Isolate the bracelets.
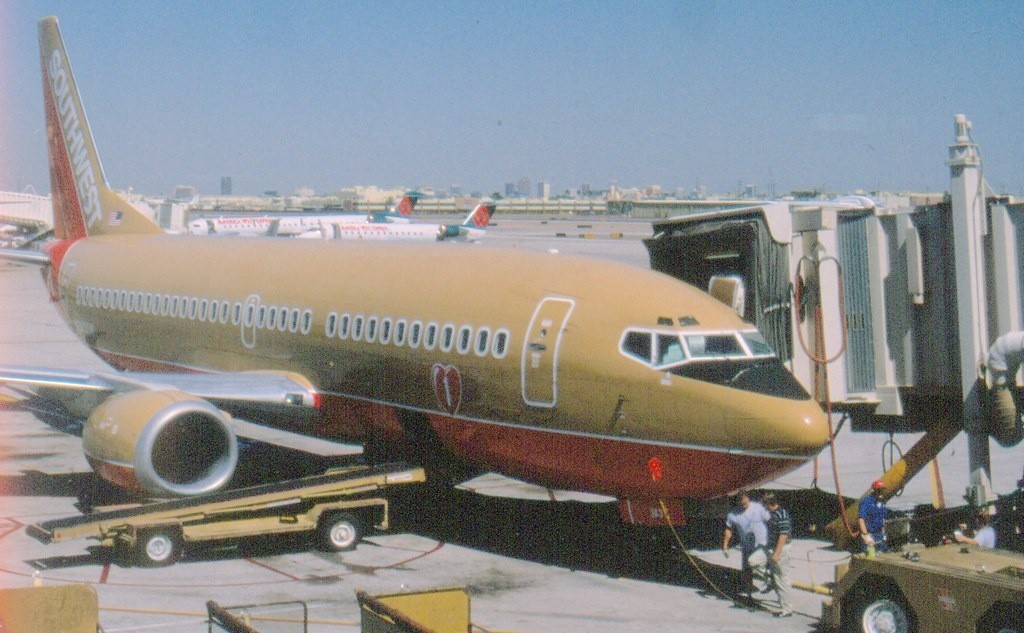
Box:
[773,553,779,556]
[860,532,867,535]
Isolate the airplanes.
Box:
[0,15,829,565]
[187,191,499,243]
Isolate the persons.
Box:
[859,481,888,555]
[76,474,94,514]
[937,504,995,548]
[722,492,792,617]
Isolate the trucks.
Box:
[25,461,426,565]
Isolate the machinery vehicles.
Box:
[813,486,1022,633]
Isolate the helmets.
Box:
[873,480,884,489]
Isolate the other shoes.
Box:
[776,610,790,617]
